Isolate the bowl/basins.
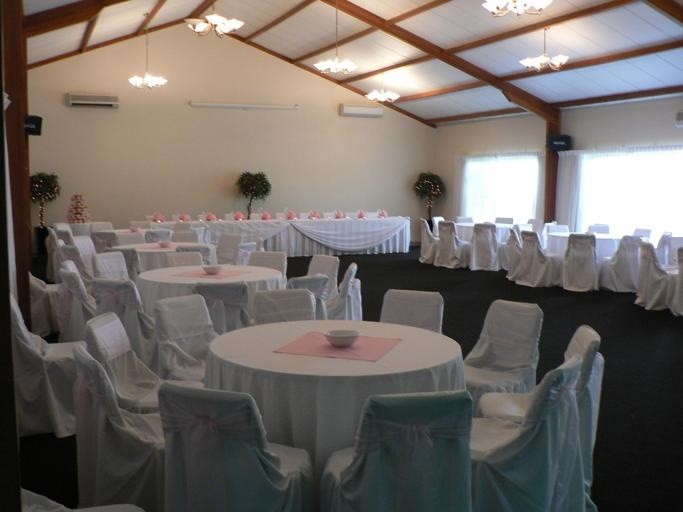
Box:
[324,329,358,347]
[201,264,221,274]
[158,241,170,248]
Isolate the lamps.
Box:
[183,0,246,40]
[518,24,570,74]
[481,1,553,17]
[364,86,400,104]
[311,1,358,77]
[126,11,169,90]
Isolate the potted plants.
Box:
[409,170,447,233]
[27,169,64,259]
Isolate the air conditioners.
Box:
[337,103,383,118]
[65,90,119,111]
